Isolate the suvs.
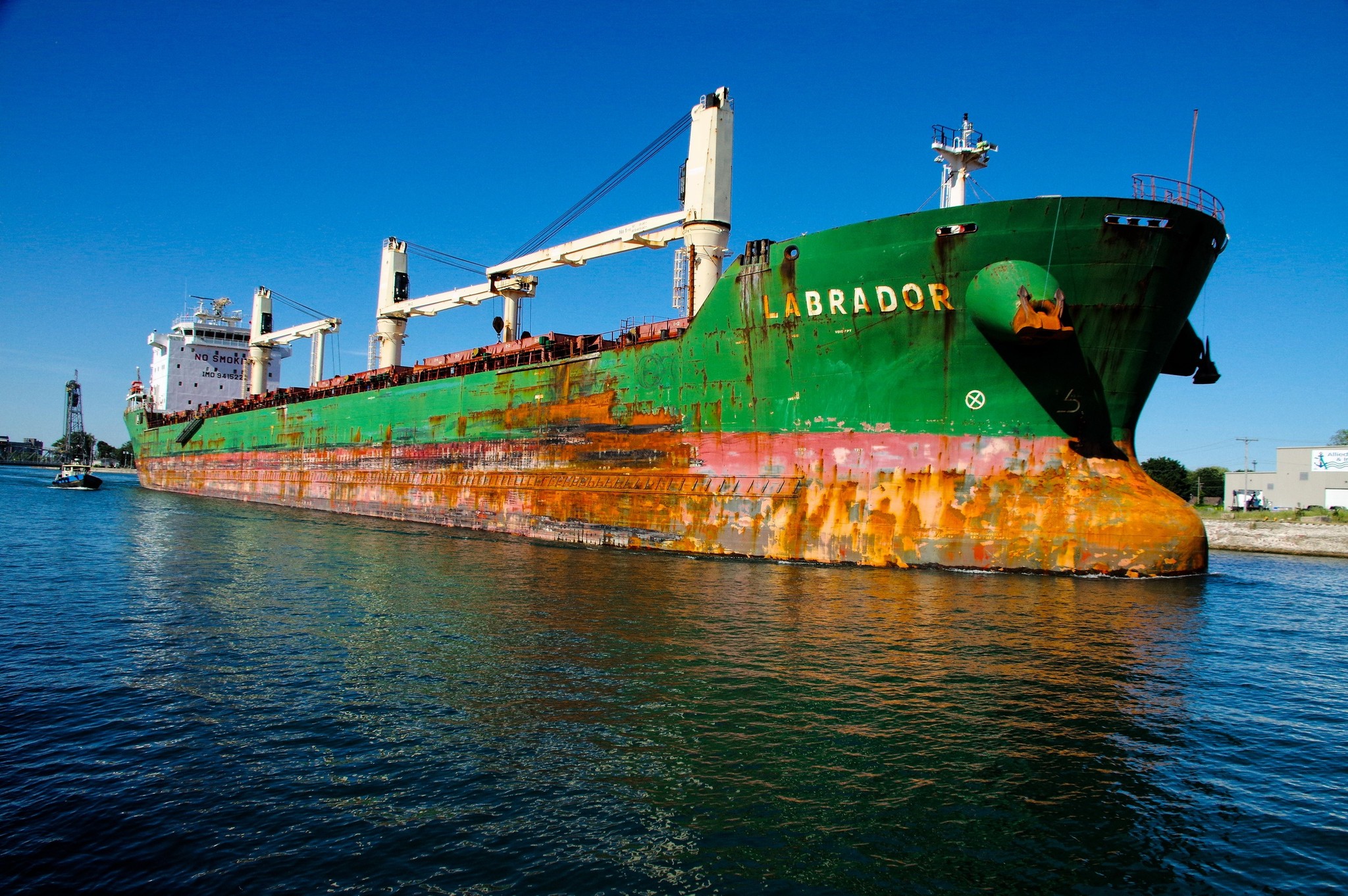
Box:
[1307,505,1347,511]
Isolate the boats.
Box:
[52,459,103,491]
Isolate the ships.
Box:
[121,85,1227,578]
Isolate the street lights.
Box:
[123,450,128,466]
